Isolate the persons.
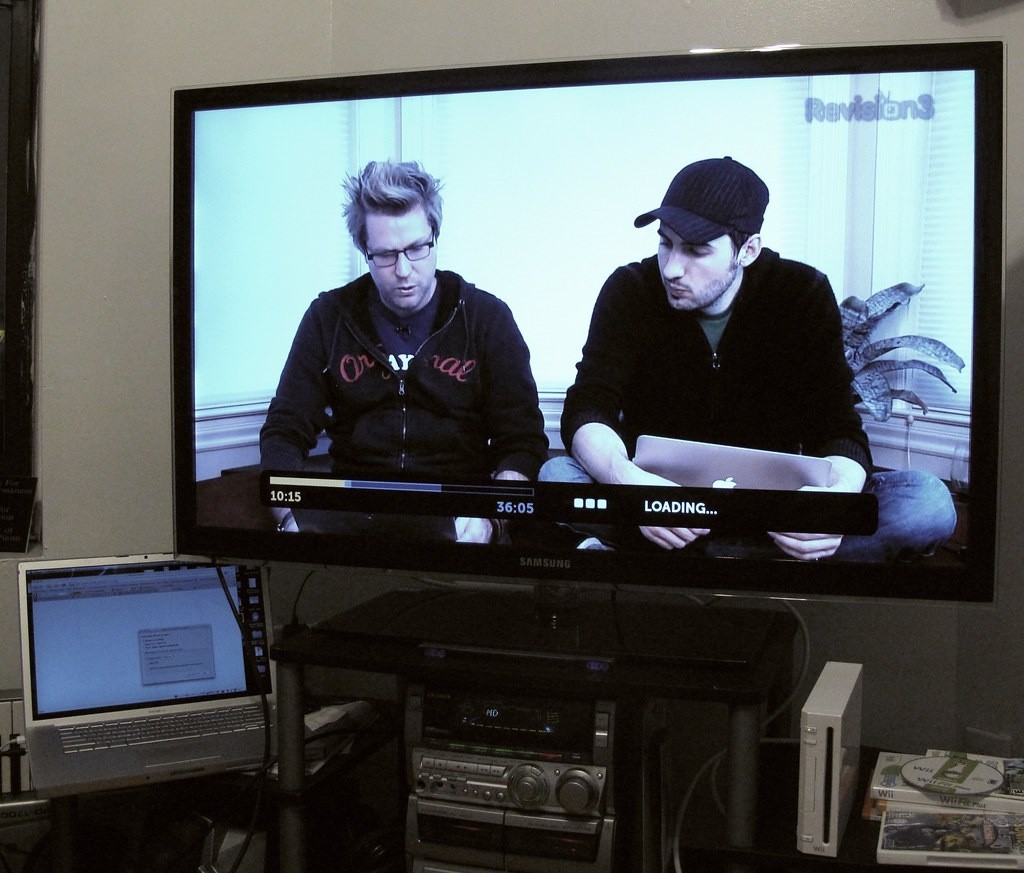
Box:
[259,161,548,544]
[534,156,957,562]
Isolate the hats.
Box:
[634,157,770,244]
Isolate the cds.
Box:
[900,757,1005,795]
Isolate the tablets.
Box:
[633,434,831,492]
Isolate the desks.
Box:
[267,595,781,873]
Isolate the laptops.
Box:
[18,551,325,802]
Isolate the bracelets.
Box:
[490,519,503,543]
[277,511,293,531]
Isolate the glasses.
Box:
[365,227,434,268]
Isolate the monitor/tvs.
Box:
[173,41,1006,665]
